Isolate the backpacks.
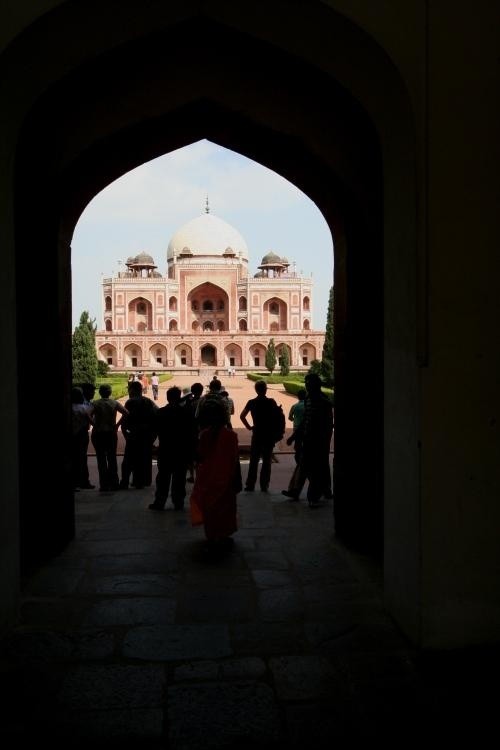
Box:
[259,403,287,444]
